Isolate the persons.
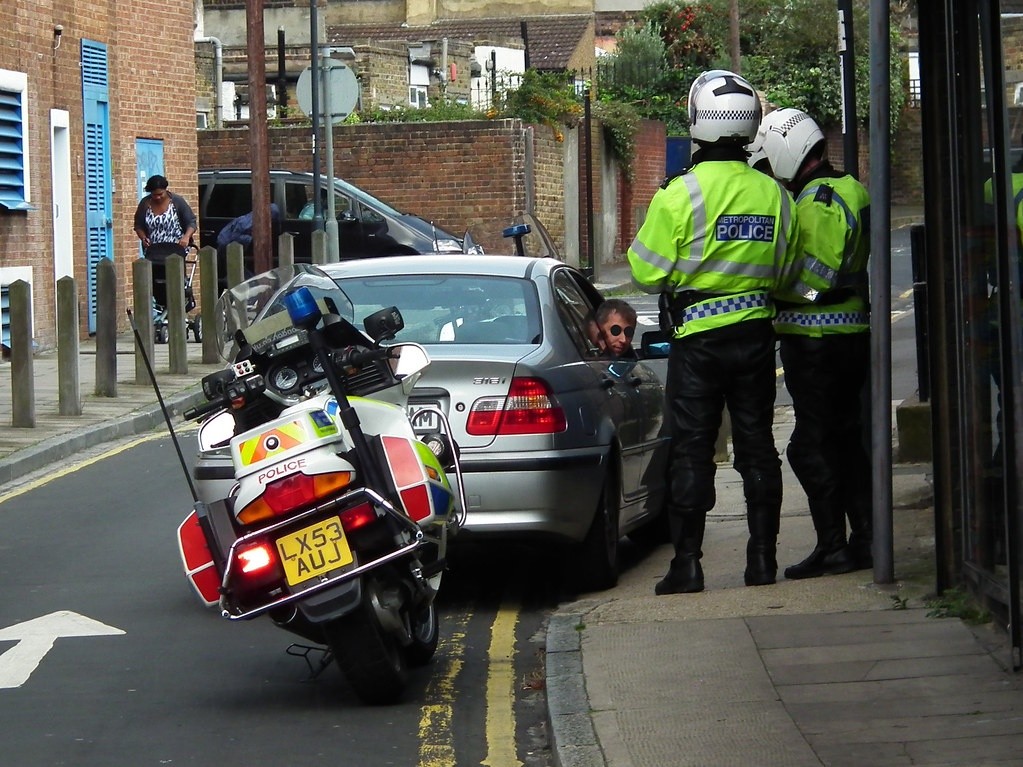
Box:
[983,107,1023,565]
[584,312,610,354]
[298,188,328,219]
[217,204,282,285]
[628,70,803,595]
[134,175,197,339]
[748,104,871,579]
[597,298,638,356]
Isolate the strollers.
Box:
[146,242,202,343]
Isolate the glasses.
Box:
[601,326,636,339]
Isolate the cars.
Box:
[227,255,670,592]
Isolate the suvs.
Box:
[197,167,484,308]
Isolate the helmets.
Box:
[688,70,761,146]
[749,106,826,181]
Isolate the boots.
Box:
[738,472,783,584]
[784,497,852,579]
[844,486,874,570]
[653,506,703,594]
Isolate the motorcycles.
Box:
[178,262,467,706]
[436,213,594,343]
